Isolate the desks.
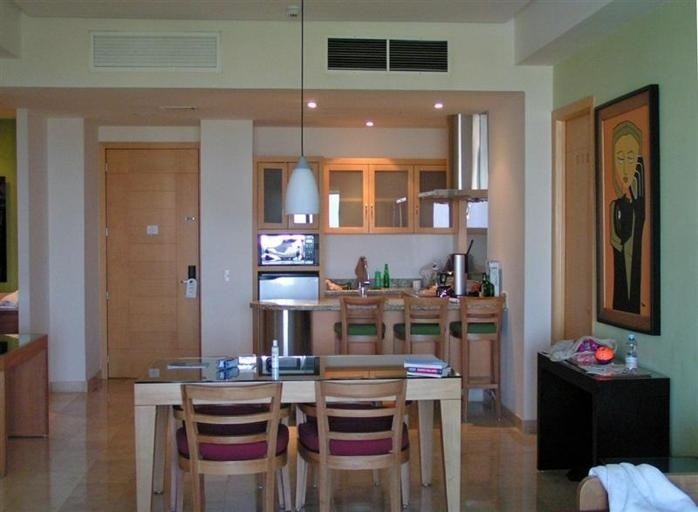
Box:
[3,332,48,479]
[536,349,671,474]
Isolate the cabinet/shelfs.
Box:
[412,160,458,235]
[323,158,414,233]
[254,155,322,230]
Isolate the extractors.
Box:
[434,113,489,203]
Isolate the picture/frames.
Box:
[597,84,659,334]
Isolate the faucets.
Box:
[359,282,370,298]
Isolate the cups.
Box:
[412,280,421,291]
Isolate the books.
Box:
[403,359,449,378]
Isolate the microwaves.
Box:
[256,234,320,267]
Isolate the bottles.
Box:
[271,339,279,380]
[384,263,390,288]
[482,272,488,297]
[624,334,639,369]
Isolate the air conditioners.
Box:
[92,32,229,71]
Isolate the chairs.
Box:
[451,294,508,420]
[298,366,408,512]
[339,292,385,352]
[392,290,451,361]
[172,384,290,512]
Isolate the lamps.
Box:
[282,2,321,216]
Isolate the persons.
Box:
[609,122,644,314]
